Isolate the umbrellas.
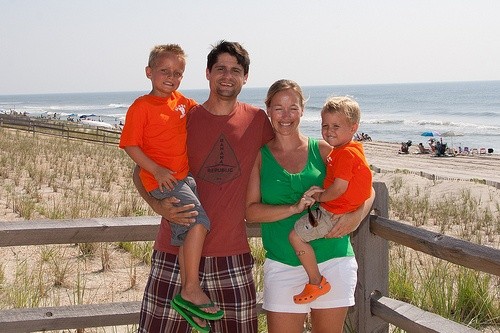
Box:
[421,131,464,147]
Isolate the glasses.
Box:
[307,205,322,227]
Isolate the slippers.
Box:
[174,294,224,320]
[170,300,210,333]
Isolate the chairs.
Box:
[418,143,494,158]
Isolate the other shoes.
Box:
[293,275,331,304]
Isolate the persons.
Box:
[119,43,225,333]
[287,96,372,303]
[138,43,276,333]
[245,79,376,333]
[361,132,442,155]
